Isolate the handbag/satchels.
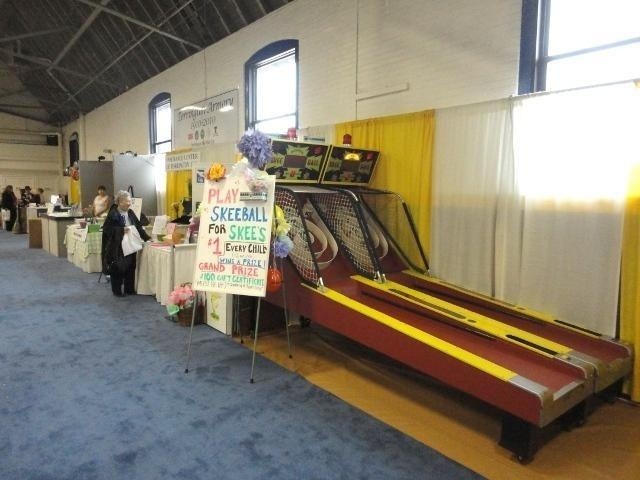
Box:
[121,225,144,257]
[1,209,11,222]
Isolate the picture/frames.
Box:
[173,88,240,150]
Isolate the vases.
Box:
[177,307,201,326]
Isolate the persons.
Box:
[101,203,120,282]
[92,184,110,218]
[1,184,18,234]
[21,185,36,205]
[37,186,47,206]
[102,188,154,298]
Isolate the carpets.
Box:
[0,230,486,480]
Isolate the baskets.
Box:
[176,282,204,327]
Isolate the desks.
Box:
[16,201,198,306]
